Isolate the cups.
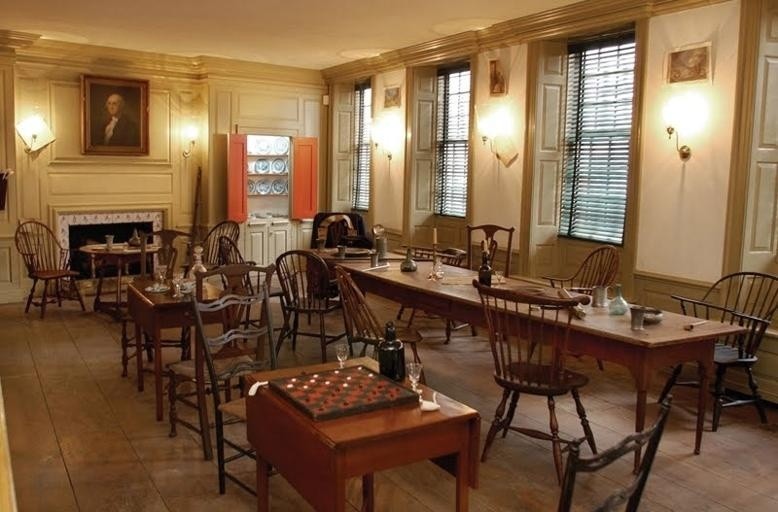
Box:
[370,253,378,268]
[315,238,327,251]
[592,285,608,306]
[376,238,387,257]
[337,245,346,259]
[630,308,645,333]
[105,235,113,250]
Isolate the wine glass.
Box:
[495,271,503,286]
[156,265,167,288]
[335,344,350,369]
[406,362,424,395]
[171,269,185,298]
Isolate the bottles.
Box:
[400,249,417,272]
[607,284,629,316]
[189,245,208,284]
[128,228,142,248]
[479,253,492,287]
[377,322,405,383]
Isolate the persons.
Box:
[97,94,138,146]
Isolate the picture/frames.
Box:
[80,74,148,156]
[662,42,712,87]
[487,57,509,97]
[382,85,403,109]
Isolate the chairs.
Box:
[16,221,86,319]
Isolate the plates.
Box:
[246,137,289,195]
[145,285,171,294]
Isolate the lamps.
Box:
[478,118,503,160]
[662,107,692,162]
[184,127,198,158]
[24,124,41,154]
[371,128,392,160]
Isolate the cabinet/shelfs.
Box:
[226,124,320,277]
